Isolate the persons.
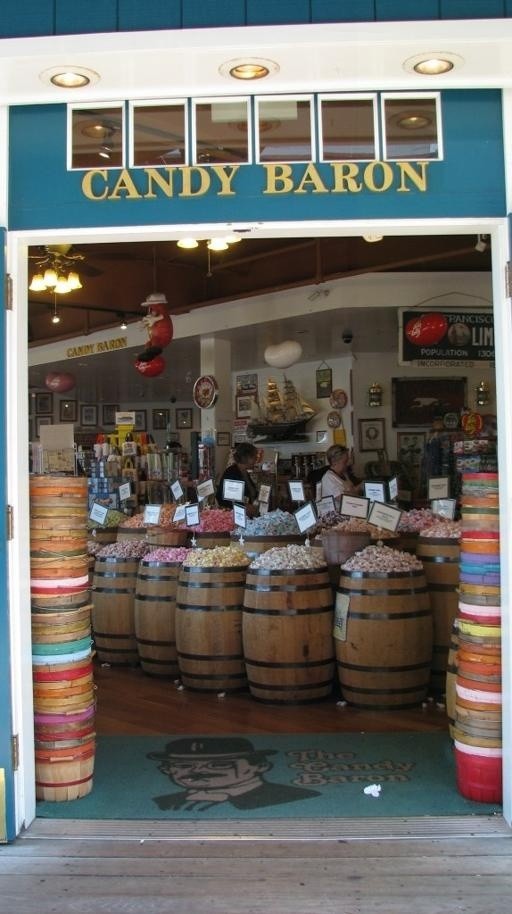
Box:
[215,442,262,520]
[320,444,367,514]
[149,736,324,816]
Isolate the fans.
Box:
[28,244,105,277]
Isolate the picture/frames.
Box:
[358,417,386,452]
[397,431,426,467]
[35,392,192,438]
[393,374,468,428]
[316,431,329,444]
[235,392,256,419]
[217,431,230,446]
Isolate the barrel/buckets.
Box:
[416,533,460,680]
[25,476,99,803]
[114,525,147,542]
[456,471,502,802]
[89,555,142,668]
[147,527,185,553]
[240,567,337,701]
[87,528,113,544]
[172,565,248,693]
[330,568,433,706]
[444,617,455,742]
[320,531,370,566]
[232,532,307,557]
[186,531,231,550]
[131,560,181,677]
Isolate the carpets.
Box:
[35,726,502,819]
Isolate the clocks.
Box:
[193,375,216,409]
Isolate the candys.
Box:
[85,502,462,574]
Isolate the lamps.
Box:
[475,380,489,406]
[29,259,83,325]
[367,382,383,407]
[120,322,127,330]
[176,238,242,277]
